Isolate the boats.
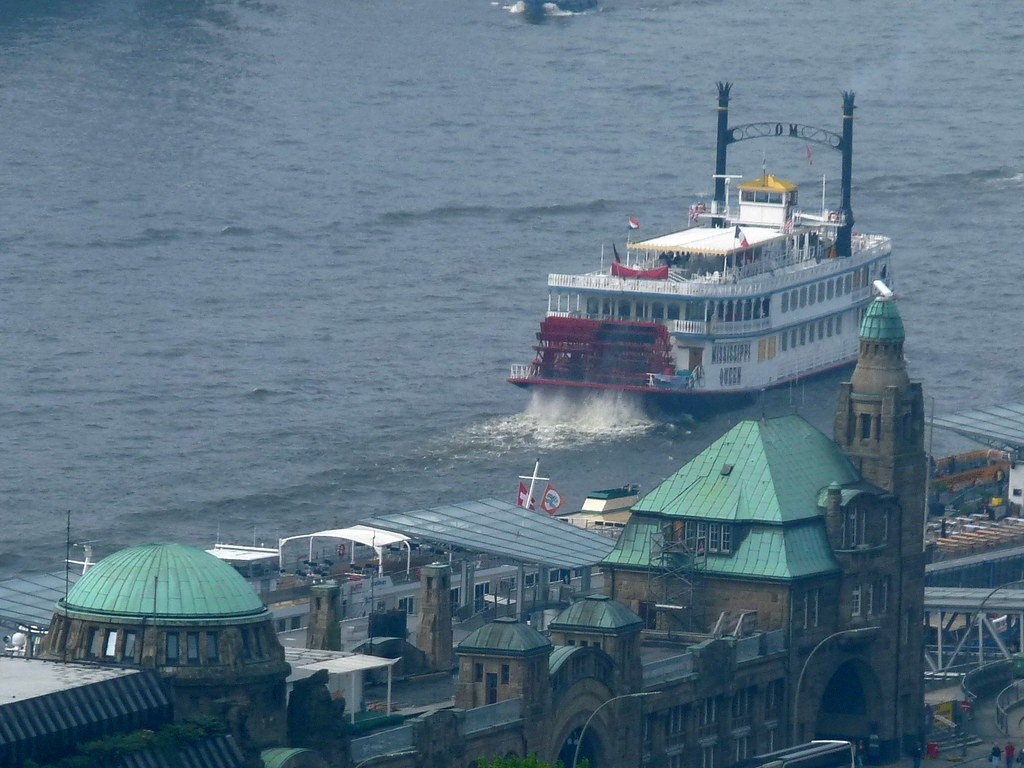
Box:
[521,0,597,26]
[503,75,898,404]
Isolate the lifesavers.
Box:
[696,202,706,214]
[828,211,840,223]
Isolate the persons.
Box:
[990,741,1016,768]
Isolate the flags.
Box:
[783,216,793,235]
[734,224,750,248]
[516,480,565,516]
[628,216,639,229]
